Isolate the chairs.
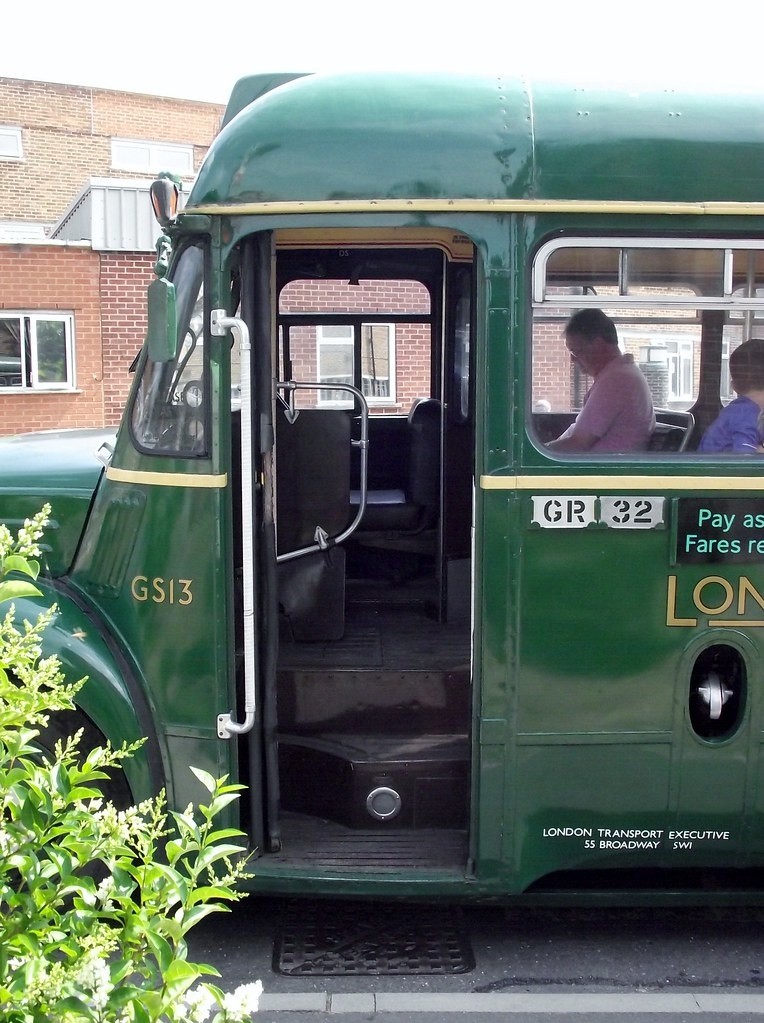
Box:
[649,407,696,452]
[351,397,448,621]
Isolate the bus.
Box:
[0,72,764,902]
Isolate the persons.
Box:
[536,400,551,412]
[544,308,656,453]
[697,339,764,453]
